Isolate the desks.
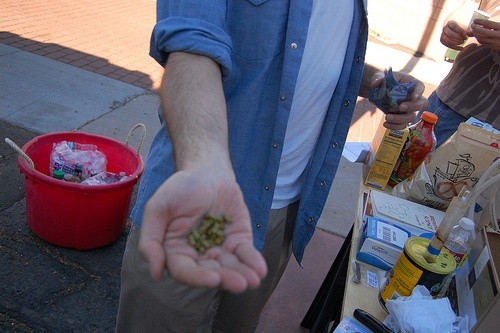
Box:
[301,170,394,333]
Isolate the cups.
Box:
[467,9,490,32]
[444,32,468,64]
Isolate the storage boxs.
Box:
[358,215,415,272]
[362,188,446,237]
[362,114,414,191]
[455,222,500,332]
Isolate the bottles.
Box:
[52,170,64,182]
[388,111,438,187]
[116,172,125,183]
[437,217,475,299]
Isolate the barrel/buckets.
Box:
[5,122,146,248]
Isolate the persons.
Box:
[415,0,500,150]
[117,0,429,333]
